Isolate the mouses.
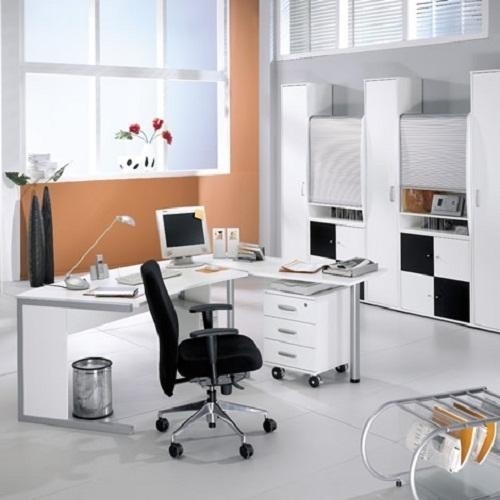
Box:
[205,265,219,271]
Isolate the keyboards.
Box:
[116,266,183,286]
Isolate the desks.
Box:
[17,254,377,434]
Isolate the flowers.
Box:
[115,117,173,145]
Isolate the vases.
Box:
[128,145,164,170]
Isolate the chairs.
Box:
[140,260,278,461]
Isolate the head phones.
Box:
[154,205,213,269]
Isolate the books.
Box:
[238,243,265,261]
[407,402,497,474]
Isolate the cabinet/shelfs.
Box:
[262,285,357,388]
[280,70,500,336]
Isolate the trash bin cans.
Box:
[72,357,113,420]
